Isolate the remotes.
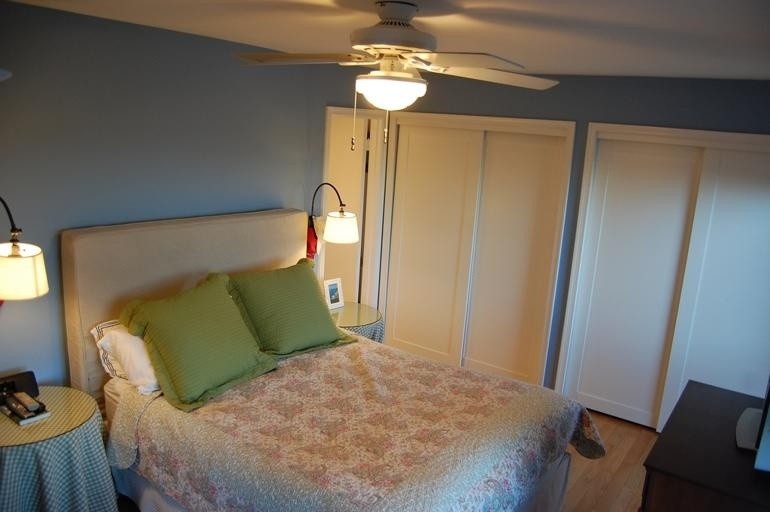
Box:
[14,392,40,411]
[6,397,35,418]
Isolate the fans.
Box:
[231,1,559,91]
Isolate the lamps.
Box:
[354,69,428,112]
[0,239,50,303]
[311,180,361,247]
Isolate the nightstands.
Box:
[330,303,384,344]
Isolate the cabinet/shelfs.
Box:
[557,113,761,430]
[378,105,570,388]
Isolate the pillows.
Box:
[114,272,282,408]
[91,318,162,395]
[230,259,359,361]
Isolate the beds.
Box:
[57,207,595,512]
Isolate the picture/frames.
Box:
[323,276,345,310]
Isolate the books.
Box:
[1,397,52,426]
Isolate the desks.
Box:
[639,379,770,511]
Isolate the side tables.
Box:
[1,382,123,512]
